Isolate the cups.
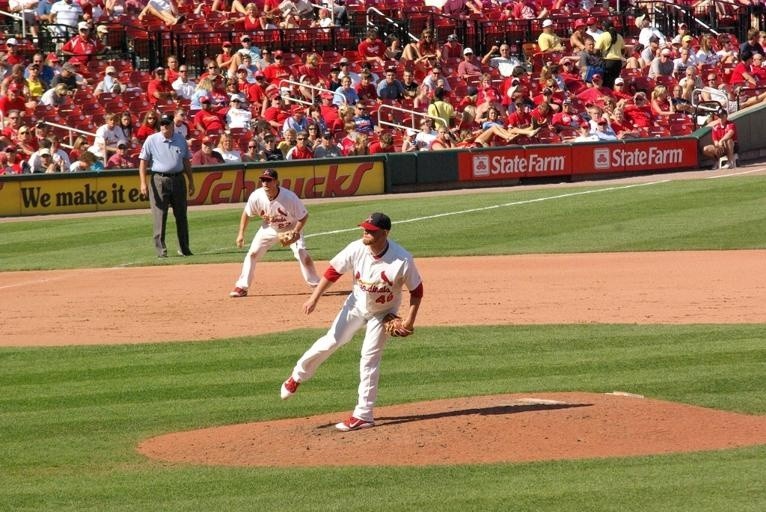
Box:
[411,141,416,145]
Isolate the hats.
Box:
[5,21,741,158]
[357,211,391,232]
[259,168,278,181]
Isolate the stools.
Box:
[718,153,739,168]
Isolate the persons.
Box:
[230,169,326,296]
[279,212,423,431]
[1,1,766,177]
[136,113,195,257]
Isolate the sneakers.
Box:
[228,286,248,298]
[280,375,301,400]
[333,415,376,433]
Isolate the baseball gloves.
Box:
[278,230,299,248]
[382,312,413,338]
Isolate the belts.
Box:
[155,172,183,177]
